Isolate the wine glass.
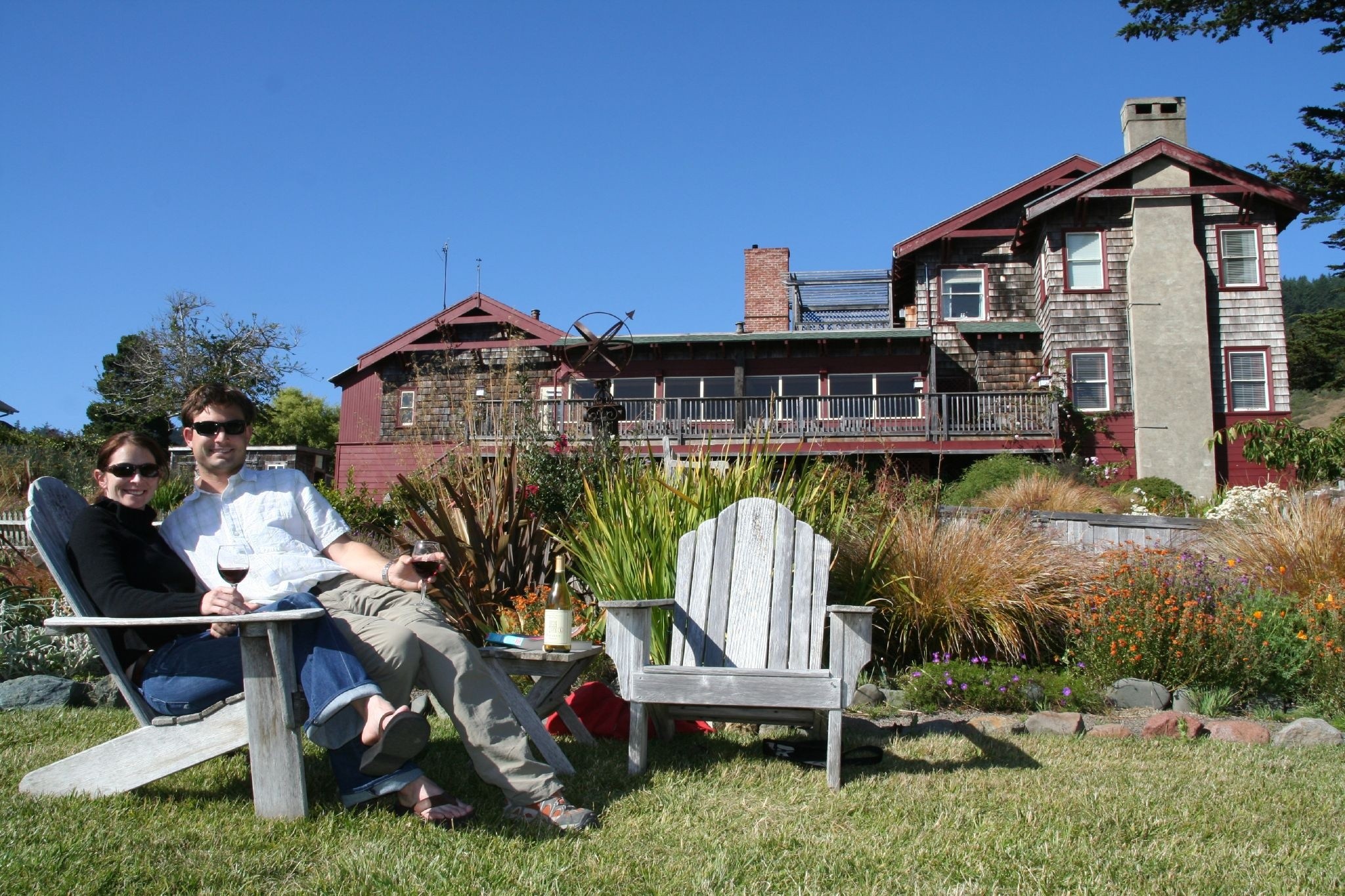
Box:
[217,544,249,589]
[409,541,440,606]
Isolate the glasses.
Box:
[102,463,160,478]
[191,419,249,436]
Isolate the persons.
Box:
[158,382,594,832]
[65,429,475,823]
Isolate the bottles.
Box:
[543,555,572,653]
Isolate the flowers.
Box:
[1028,372,1041,383]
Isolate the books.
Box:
[485,623,593,650]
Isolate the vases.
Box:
[1031,381,1040,389]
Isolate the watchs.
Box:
[382,562,394,588]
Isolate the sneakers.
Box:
[502,790,600,835]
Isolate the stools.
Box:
[477,642,602,775]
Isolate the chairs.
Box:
[19,476,325,820]
[599,498,875,793]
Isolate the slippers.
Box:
[357,710,430,778]
[394,790,477,823]
